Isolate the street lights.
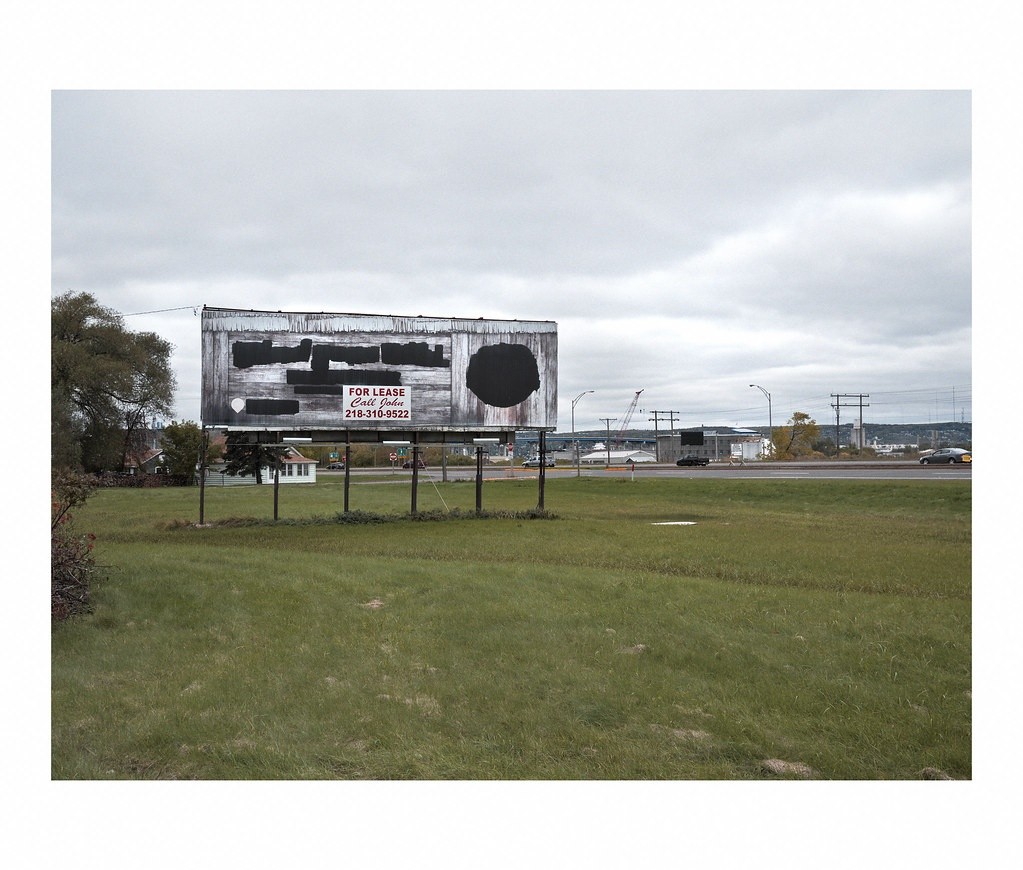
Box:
[571,390,595,467]
[749,384,772,456]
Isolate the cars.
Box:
[521,456,555,468]
[326,462,345,469]
[919,447,972,464]
[402,459,425,469]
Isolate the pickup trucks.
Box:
[676,455,709,466]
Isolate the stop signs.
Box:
[390,452,397,461]
